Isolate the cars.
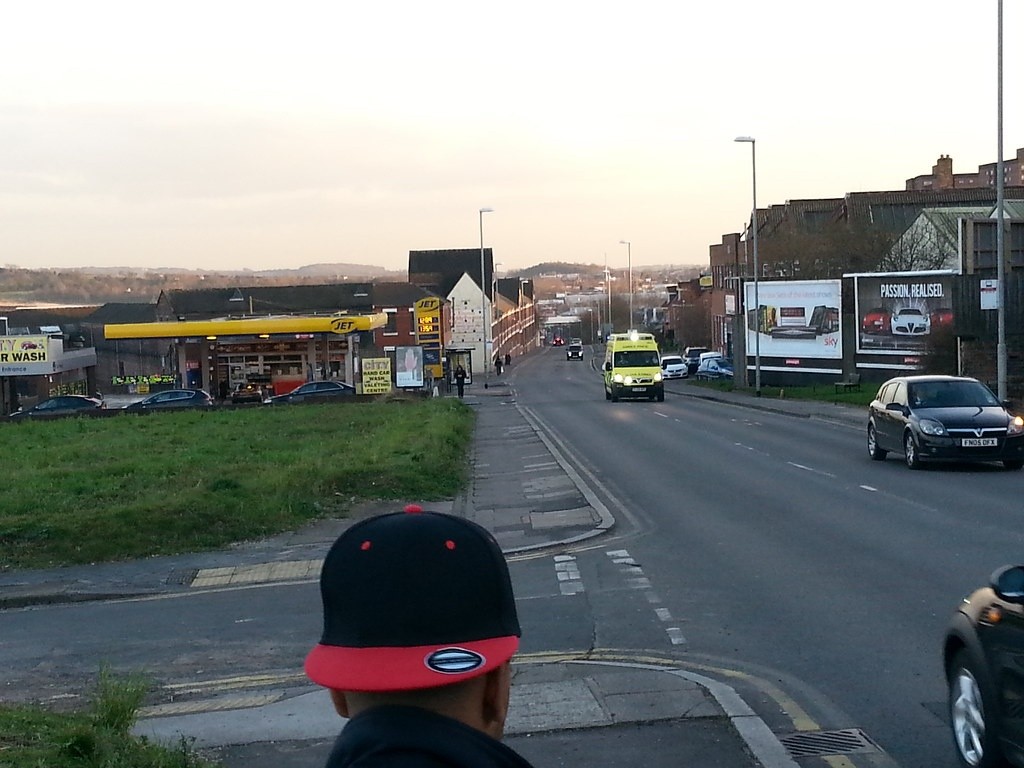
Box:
[694,351,733,383]
[264,380,382,406]
[943,563,1024,768]
[867,374,1024,469]
[553,338,564,346]
[863,308,954,335]
[122,388,214,410]
[661,356,688,379]
[10,394,107,418]
[572,338,582,345]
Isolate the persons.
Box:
[455,365,465,398]
[618,355,631,365]
[303,505,530,768]
[495,356,502,375]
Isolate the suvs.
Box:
[566,345,584,360]
[682,347,710,371]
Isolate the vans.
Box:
[603,332,667,402]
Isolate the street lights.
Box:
[620,240,633,332]
[495,262,503,358]
[596,301,601,345]
[480,207,494,389]
[521,280,529,355]
[602,270,612,335]
[735,135,761,397]
[588,309,593,344]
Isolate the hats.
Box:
[303,504,520,690]
